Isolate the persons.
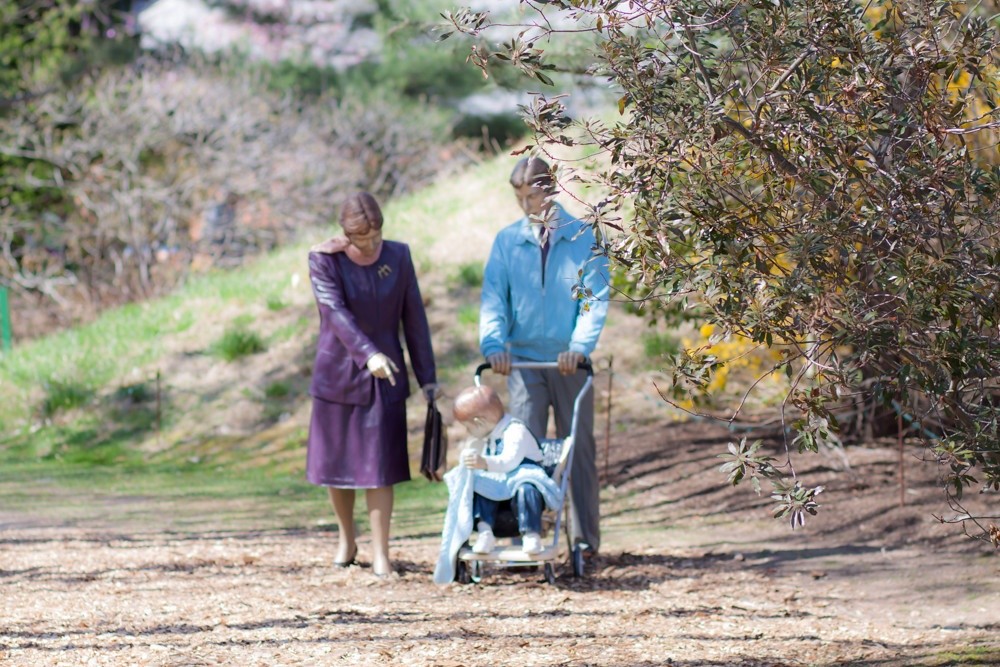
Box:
[305,192,439,578]
[453,385,548,556]
[478,155,611,568]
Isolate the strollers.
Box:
[455,362,594,584]
